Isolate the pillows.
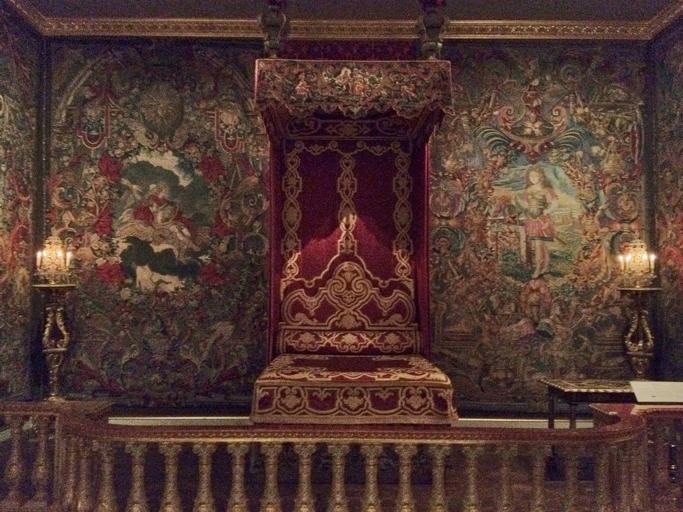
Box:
[275,329,421,356]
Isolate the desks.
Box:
[68,400,115,427]
[537,375,648,428]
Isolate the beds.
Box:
[249,58,462,426]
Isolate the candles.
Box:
[36,249,72,267]
[619,255,657,269]
